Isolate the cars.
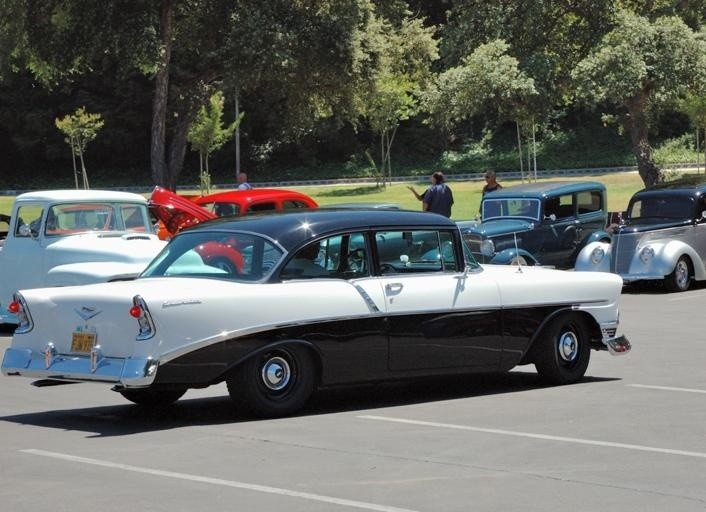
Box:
[0,193,205,241]
[237,202,478,278]
[574,176,706,292]
[150,185,319,274]
[0,188,231,325]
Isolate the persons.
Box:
[406,184,430,211]
[479,170,509,216]
[426,170,454,217]
[237,172,250,190]
[284,240,328,277]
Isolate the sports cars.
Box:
[0,207,632,417]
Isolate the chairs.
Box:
[278,237,320,275]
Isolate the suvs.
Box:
[422,181,612,272]
